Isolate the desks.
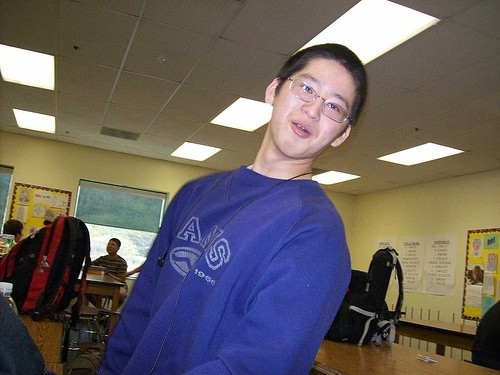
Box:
[74,270,127,341]
[394,318,478,356]
[19,312,66,375]
[312,339,500,375]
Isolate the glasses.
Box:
[288,76,356,125]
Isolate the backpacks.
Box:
[323,246,404,346]
[0,215,92,326]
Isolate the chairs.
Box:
[57,286,128,375]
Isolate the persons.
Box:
[125,264,143,276]
[84,238,129,312]
[2,219,24,246]
[94,44,368,375]
[471,299,500,370]
